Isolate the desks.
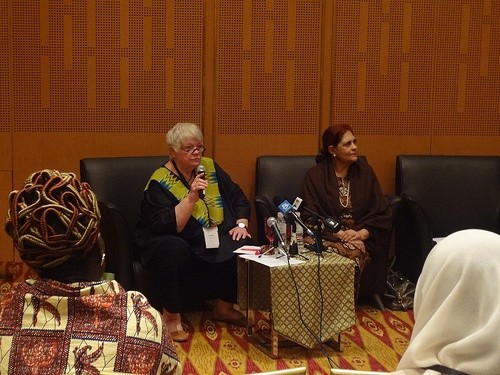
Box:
[244,248,356,359]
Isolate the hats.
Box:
[4,168,102,271]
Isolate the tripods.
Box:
[258,215,332,260]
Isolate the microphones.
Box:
[197,166,206,199]
[267,193,341,253]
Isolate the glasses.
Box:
[181,144,207,154]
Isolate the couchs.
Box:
[395,155,500,282]
[254,155,402,304]
[79,155,221,312]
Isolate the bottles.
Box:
[290,212,304,254]
[275,212,287,250]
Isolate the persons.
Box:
[393,230,500,375]
[300,124,393,308]
[0,169,183,375]
[135,123,252,328]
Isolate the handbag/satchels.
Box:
[383,255,416,309]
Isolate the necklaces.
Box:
[336,170,350,207]
[189,171,194,183]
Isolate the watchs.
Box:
[235,223,248,231]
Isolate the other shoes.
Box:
[169,328,188,341]
[213,307,247,327]
